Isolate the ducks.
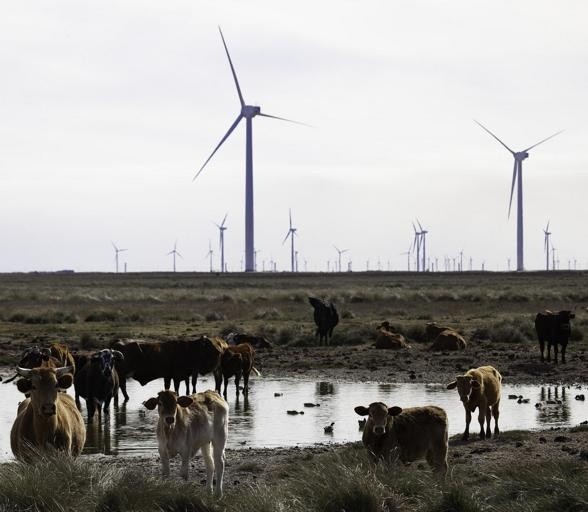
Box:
[323,422,335,433]
[516,395,530,403]
[574,393,584,400]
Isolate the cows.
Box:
[143,389,231,502]
[10,364,87,465]
[308,297,339,346]
[375,321,467,352]
[354,402,449,488]
[2,335,261,425]
[446,365,502,441]
[534,310,575,366]
